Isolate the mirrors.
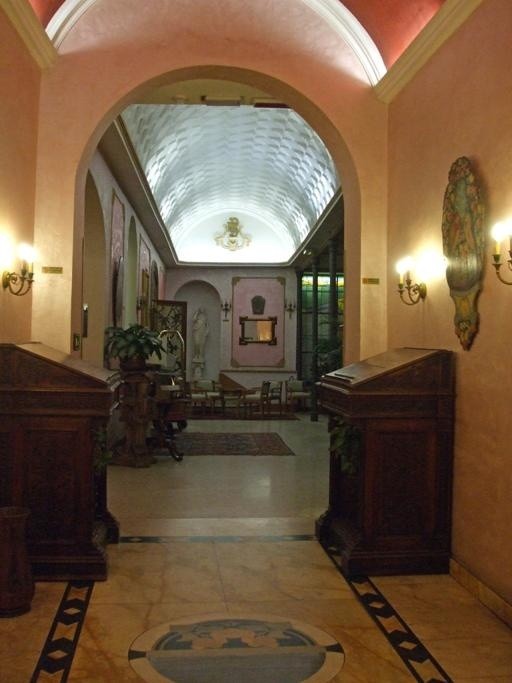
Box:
[238,315,278,345]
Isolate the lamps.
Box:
[284,295,296,317]
[3,242,36,297]
[492,219,511,285]
[214,216,252,250]
[396,259,426,305]
[221,296,232,321]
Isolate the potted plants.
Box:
[106,325,166,372]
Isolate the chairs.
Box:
[171,372,315,421]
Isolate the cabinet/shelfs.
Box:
[0,339,127,582]
[315,343,457,582]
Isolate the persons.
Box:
[191,305,210,360]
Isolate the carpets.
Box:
[157,431,295,456]
